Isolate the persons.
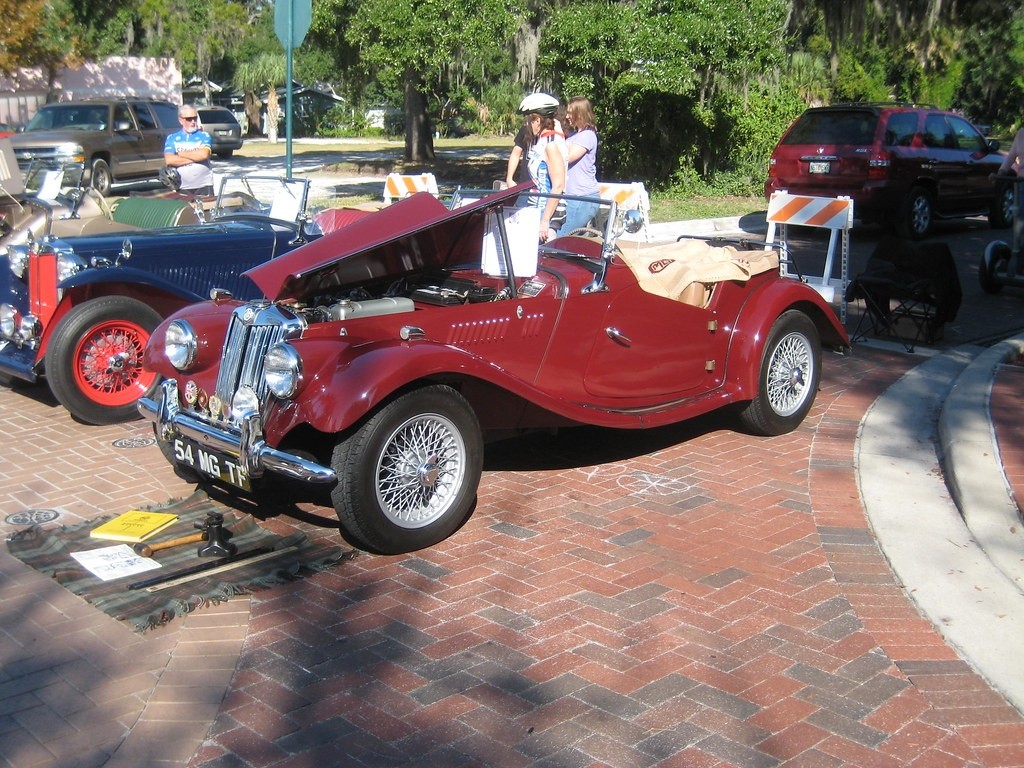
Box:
[989,126,1024,276]
[507,93,600,245]
[164,105,215,197]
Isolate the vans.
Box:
[192,105,245,157]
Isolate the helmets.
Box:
[516,93,561,117]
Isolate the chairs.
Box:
[613,239,717,302]
[108,196,200,232]
[849,237,951,354]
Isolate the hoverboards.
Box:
[978,174,1023,293]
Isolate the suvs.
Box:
[10,94,204,196]
[764,100,1021,239]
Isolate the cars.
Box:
[0,171,853,558]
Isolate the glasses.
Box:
[180,116,198,122]
